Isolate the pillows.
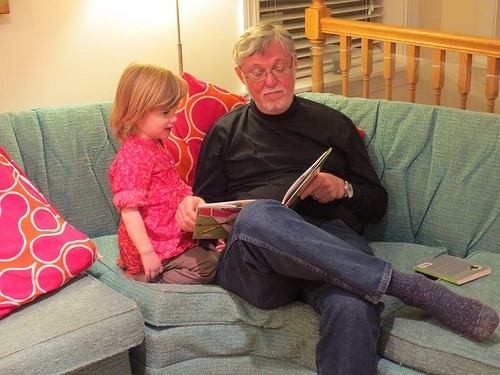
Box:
[0,144,104,321]
[162,73,250,191]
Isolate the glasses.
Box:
[239,57,293,82]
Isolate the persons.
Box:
[193,20,499,375]
[108,62,220,285]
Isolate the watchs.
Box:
[343,180,354,197]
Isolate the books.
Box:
[193,147,331,239]
[413,254,492,286]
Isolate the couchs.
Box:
[0,91,500,375]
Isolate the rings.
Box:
[315,194,321,200]
